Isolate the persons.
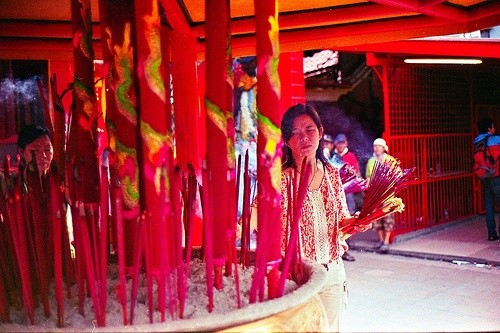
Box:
[473,118,500,242]
[223,104,372,332]
[365,137,404,252]
[8,124,60,206]
[313,132,362,262]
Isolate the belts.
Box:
[320,257,342,272]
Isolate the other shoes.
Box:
[488,235,499,241]
[374,239,384,249]
[379,243,388,251]
[347,237,353,246]
[343,252,355,262]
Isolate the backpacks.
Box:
[473,134,500,178]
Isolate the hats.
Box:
[335,134,347,143]
[324,135,334,142]
[373,138,388,153]
